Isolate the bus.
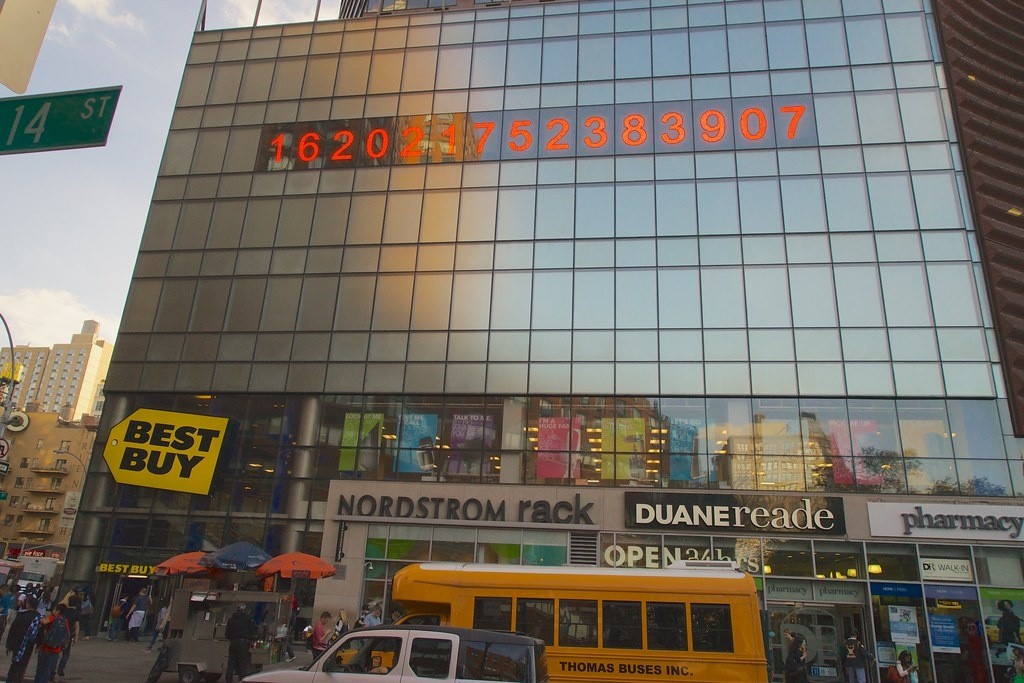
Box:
[334,557,771,683]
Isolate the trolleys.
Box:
[160,584,295,683]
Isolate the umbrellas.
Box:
[256,553,336,595]
[153,551,228,590]
[197,541,273,590]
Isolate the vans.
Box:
[235,624,549,683]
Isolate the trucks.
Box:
[17,556,60,594]
[0,558,24,591]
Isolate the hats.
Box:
[10,584,21,593]
[235,603,247,611]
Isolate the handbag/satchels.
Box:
[886,661,905,683]
[306,634,314,650]
[80,599,90,616]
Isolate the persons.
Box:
[286,611,297,663]
[391,611,400,622]
[843,629,872,683]
[0,582,96,683]
[1009,648,1024,683]
[996,599,1023,658]
[895,650,917,683]
[782,629,810,683]
[107,588,148,642]
[224,603,259,683]
[143,591,183,652]
[304,609,349,660]
[368,649,399,674]
[353,605,384,630]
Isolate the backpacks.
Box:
[22,592,36,609]
[43,615,67,648]
[337,618,349,634]
[111,600,127,618]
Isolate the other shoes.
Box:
[82,636,89,641]
[142,647,151,652]
[57,667,64,676]
[286,657,296,662]
